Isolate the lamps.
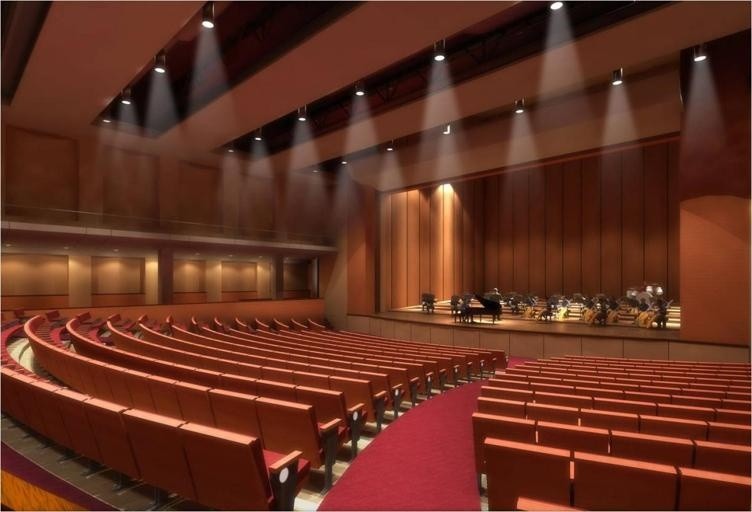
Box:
[227,127,263,153]
[298,103,307,122]
[434,38,446,61]
[694,42,707,62]
[312,136,395,172]
[201,1,214,28]
[514,97,526,114]
[611,67,623,86]
[354,78,365,96]
[120,87,133,105]
[151,47,168,76]
[441,124,451,134]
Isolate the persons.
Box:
[522,295,660,329]
[490,287,503,321]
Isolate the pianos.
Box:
[470,290,501,324]
